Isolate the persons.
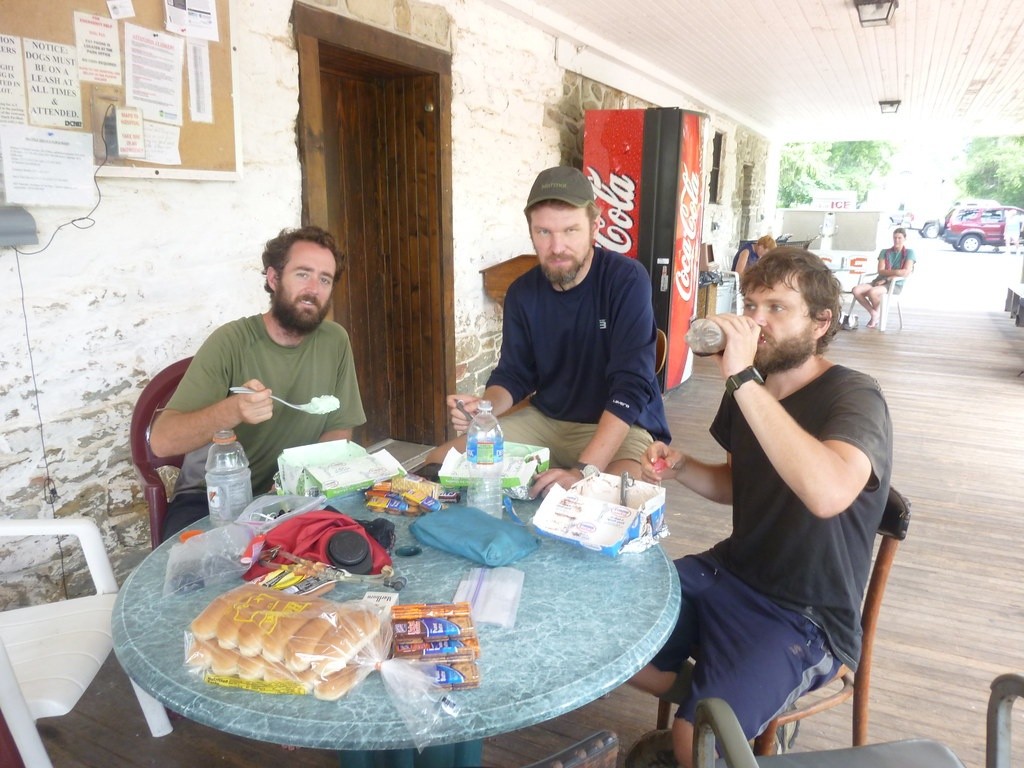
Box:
[731,234,777,278]
[620,249,893,768]
[852,227,917,330]
[422,169,673,488]
[150,226,367,544]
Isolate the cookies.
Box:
[390,615,481,692]
[366,476,441,516]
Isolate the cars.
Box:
[886,200,948,240]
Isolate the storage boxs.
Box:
[276,439,408,500]
[438,440,550,489]
[533,471,667,558]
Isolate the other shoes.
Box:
[662,656,694,704]
[622,730,677,768]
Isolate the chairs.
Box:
[848,272,910,331]
[693,672,1024,768]
[656,485,911,756]
[130,356,195,550]
[523,729,619,768]
[0,519,174,768]
[715,248,746,316]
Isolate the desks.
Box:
[110,477,681,768]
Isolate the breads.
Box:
[186,584,394,700]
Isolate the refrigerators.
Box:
[581,106,712,399]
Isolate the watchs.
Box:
[725,365,765,396]
[570,462,600,481]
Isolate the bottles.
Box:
[465,401,503,522]
[684,318,766,357]
[204,429,254,526]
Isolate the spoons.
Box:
[228,386,340,414]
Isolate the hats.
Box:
[525,166,593,214]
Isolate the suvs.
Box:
[950,200,1003,221]
[942,205,1024,253]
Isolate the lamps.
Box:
[879,101,901,113]
[854,0,899,28]
